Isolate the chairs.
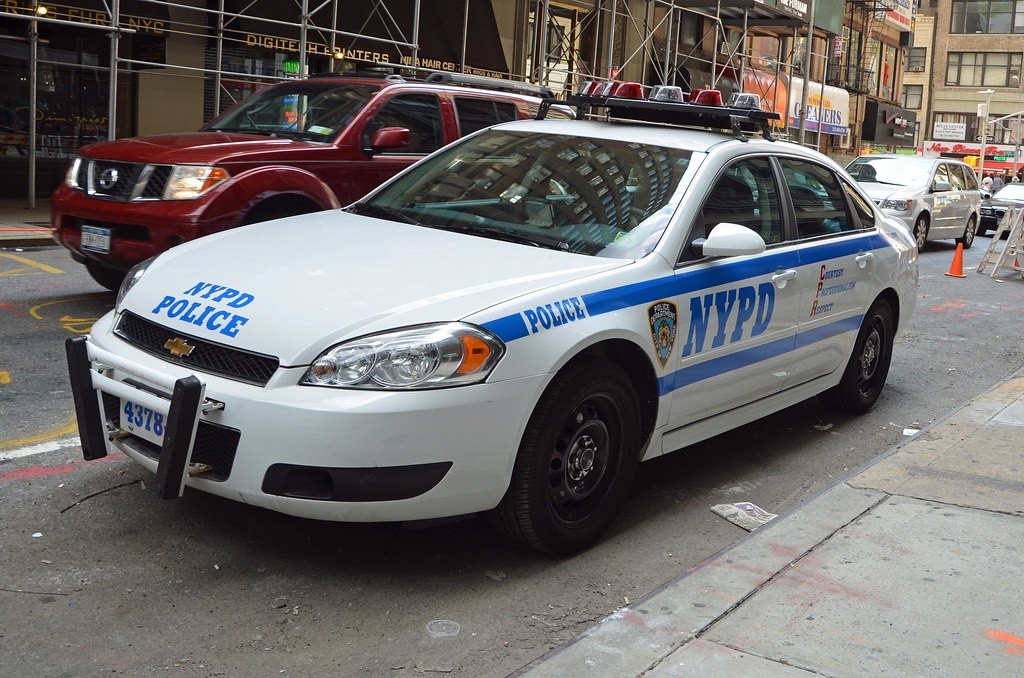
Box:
[758,186,827,237]
[703,173,754,254]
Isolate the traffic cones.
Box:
[943,242,967,278]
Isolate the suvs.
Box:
[51,67,576,296]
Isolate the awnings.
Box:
[974,160,1024,177]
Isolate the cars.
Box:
[975,182,1024,240]
[63,80,922,557]
[820,152,983,254]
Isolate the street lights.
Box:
[976,88,995,188]
[1012,74,1022,180]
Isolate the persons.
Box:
[983,174,993,186]
[992,173,1004,193]
[1012,176,1019,184]
[382,106,420,152]
[983,181,990,192]
